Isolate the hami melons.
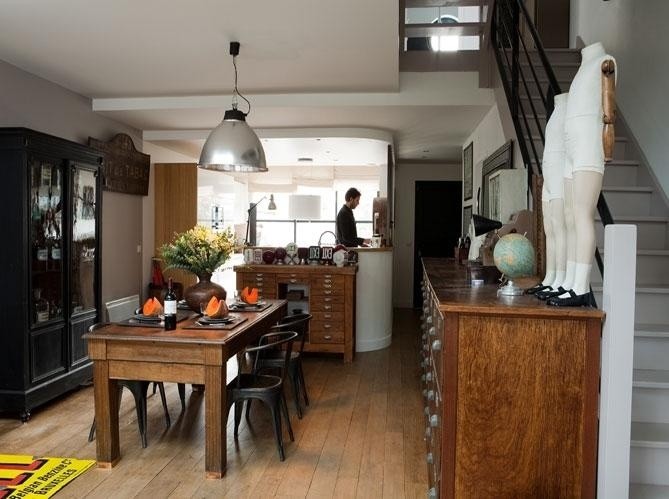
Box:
[143,297,163,316]
[241,286,259,305]
[206,296,229,317]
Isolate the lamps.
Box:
[196,42,269,174]
[243,194,277,246]
[471,214,502,240]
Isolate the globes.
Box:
[492,228,535,297]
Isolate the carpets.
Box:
[0,454,97,499]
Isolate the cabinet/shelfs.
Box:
[0,126,105,423]
[233,264,358,365]
[418,255,606,498]
[242,246,395,353]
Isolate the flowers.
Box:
[152,223,247,274]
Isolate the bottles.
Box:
[164,278,177,330]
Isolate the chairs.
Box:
[88,321,171,448]
[225,330,297,462]
[245,313,311,421]
[135,306,202,412]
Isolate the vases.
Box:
[183,273,227,314]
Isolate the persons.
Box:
[538,40,618,305]
[334,187,374,247]
[525,92,568,298]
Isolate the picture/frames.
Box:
[462,141,473,201]
[462,204,473,239]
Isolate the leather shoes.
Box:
[537,286,569,301]
[547,289,591,306]
[533,285,553,297]
[526,282,550,294]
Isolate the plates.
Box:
[133,314,162,322]
[199,317,234,324]
